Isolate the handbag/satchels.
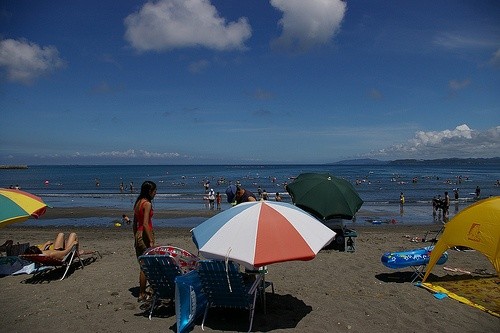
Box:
[259,193,264,201]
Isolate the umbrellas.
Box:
[0,188,48,229]
[286,173,364,220]
[189,198,337,319]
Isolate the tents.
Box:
[421,196,500,284]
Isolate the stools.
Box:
[410,263,425,283]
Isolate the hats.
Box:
[225,184,237,203]
[210,189,213,192]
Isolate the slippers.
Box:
[138,291,151,301]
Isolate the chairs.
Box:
[0,255,28,275]
[138,255,186,321]
[19,243,85,283]
[326,222,356,253]
[196,258,275,333]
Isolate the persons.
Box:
[122,214,131,224]
[412,177,418,184]
[8,184,20,190]
[400,192,404,205]
[201,175,295,207]
[24,233,84,260]
[453,188,459,199]
[119,181,134,191]
[476,186,480,198]
[133,181,157,301]
[433,191,449,215]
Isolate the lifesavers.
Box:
[381,245,448,268]
[142,245,199,273]
